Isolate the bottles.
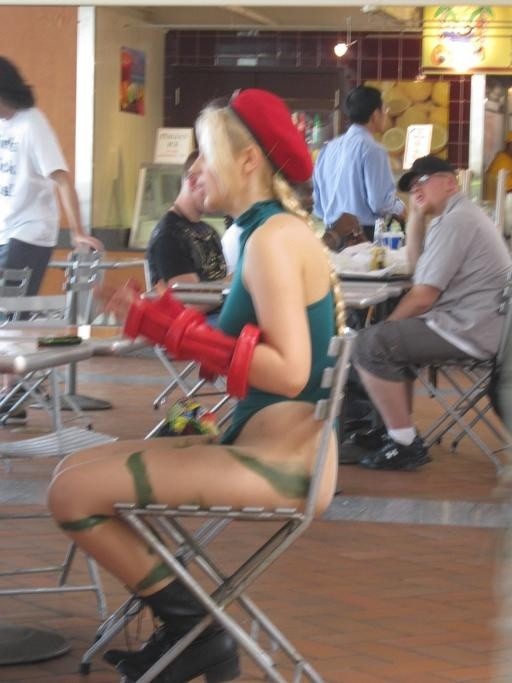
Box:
[290,108,324,146]
[470,171,482,206]
[165,395,219,436]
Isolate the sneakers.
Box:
[350,430,431,469]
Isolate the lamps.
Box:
[422,4,512,75]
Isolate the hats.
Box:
[230,88,312,182]
[399,155,454,191]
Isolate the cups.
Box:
[379,231,405,249]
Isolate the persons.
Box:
[0,59,104,426]
[47,87,347,683]
[148,88,512,472]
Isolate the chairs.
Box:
[75,322,358,683]
[1,338,121,621]
[1,252,510,476]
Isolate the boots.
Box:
[103,577,239,683]
